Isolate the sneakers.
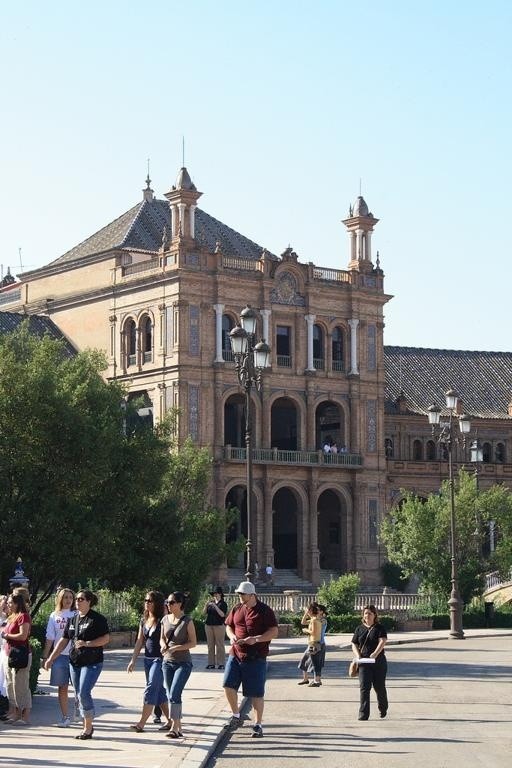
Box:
[57,716,71,728]
[223,716,245,733]
[250,725,263,738]
[153,716,161,724]
[307,680,322,687]
[298,680,309,685]
[205,665,216,669]
[218,665,225,669]
[73,705,82,723]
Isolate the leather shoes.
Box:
[234,581,258,595]
[75,726,95,740]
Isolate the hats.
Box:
[208,586,225,598]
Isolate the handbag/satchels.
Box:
[348,660,360,678]
[69,646,100,667]
[307,640,322,657]
[8,644,29,669]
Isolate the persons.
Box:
[44,587,111,740]
[158,589,198,738]
[265,563,274,587]
[221,580,279,738]
[41,587,79,727]
[0,581,34,726]
[202,585,228,669]
[297,601,323,687]
[323,441,348,454]
[253,561,260,585]
[306,604,328,686]
[127,590,172,733]
[350,603,390,721]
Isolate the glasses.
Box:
[75,597,88,602]
[166,600,181,605]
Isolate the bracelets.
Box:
[86,640,91,647]
[42,657,47,661]
[47,658,54,664]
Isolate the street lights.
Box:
[227,303,272,584]
[466,437,486,553]
[427,383,471,641]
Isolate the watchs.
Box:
[254,636,260,643]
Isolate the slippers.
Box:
[4,719,17,724]
[179,732,184,737]
[159,725,171,731]
[13,720,33,727]
[165,731,178,739]
[144,598,153,605]
[130,724,146,733]
